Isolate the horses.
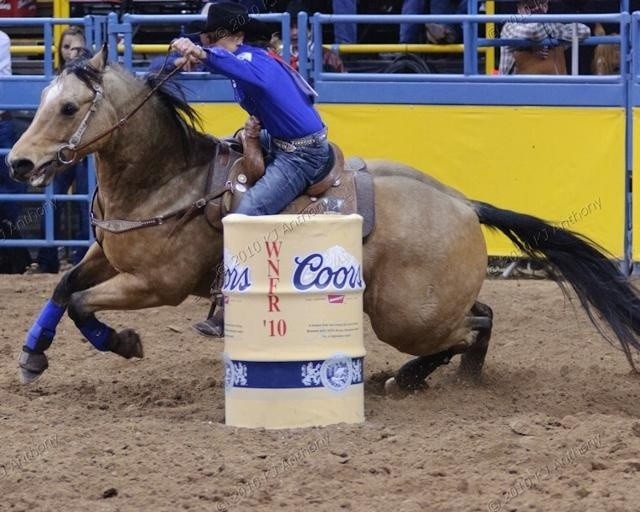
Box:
[4,42,639,386]
[588,22,622,76]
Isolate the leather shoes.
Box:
[193,308,224,338]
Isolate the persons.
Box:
[0,30,41,275]
[172,2,330,336]
[33,23,99,273]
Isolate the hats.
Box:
[183,0,250,36]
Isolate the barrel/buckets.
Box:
[220,211,364,428]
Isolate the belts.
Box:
[272,126,328,152]
